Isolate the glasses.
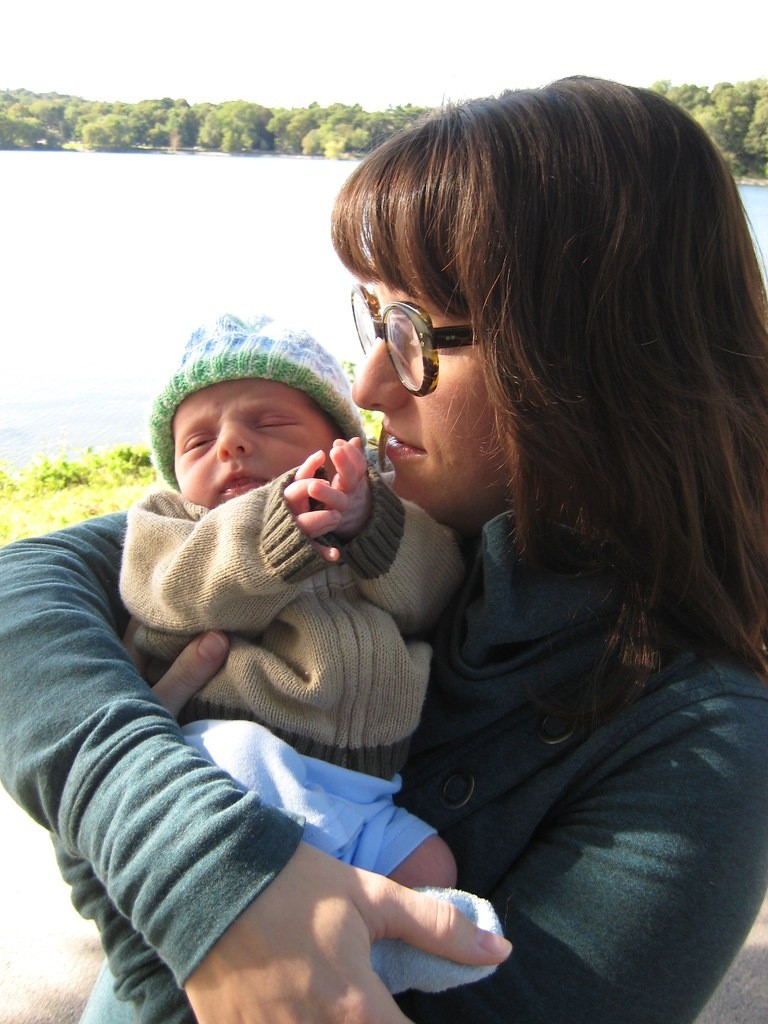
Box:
[347,285,485,390]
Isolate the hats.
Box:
[150,317,368,497]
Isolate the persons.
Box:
[2,63,768,1024]
[115,312,506,997]
